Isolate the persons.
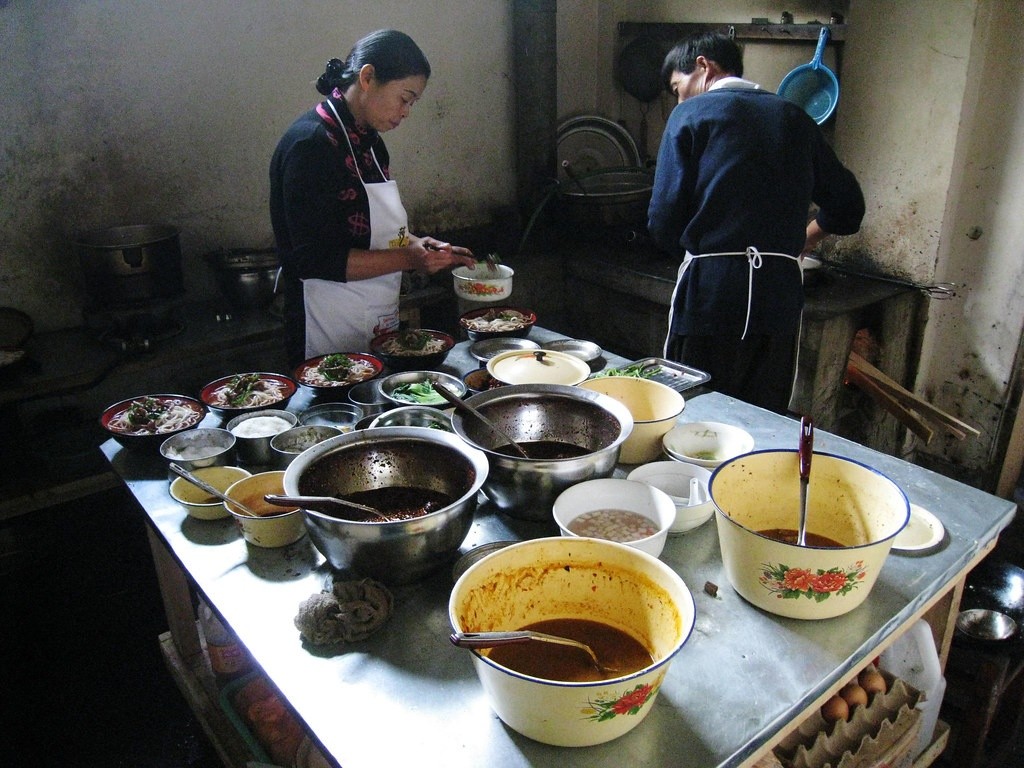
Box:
[648,29,865,417]
[269,27,477,373]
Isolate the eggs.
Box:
[822,671,885,722]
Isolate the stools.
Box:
[944,646,1024,768]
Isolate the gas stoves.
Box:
[0,300,289,404]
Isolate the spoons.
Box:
[670,478,702,508]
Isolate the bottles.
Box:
[781,11,790,24]
[829,15,835,24]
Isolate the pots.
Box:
[616,38,669,154]
[203,247,287,307]
[544,160,657,226]
[72,223,183,306]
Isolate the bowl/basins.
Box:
[291,351,385,399]
[168,465,254,520]
[707,449,911,620]
[378,370,468,407]
[662,422,755,473]
[353,412,385,430]
[451,383,634,521]
[461,367,511,395]
[198,372,298,425]
[98,393,207,453]
[368,328,456,371]
[553,478,678,558]
[225,409,298,464]
[269,425,344,466]
[457,306,537,342]
[282,426,490,586]
[451,263,514,302]
[626,461,715,533]
[574,376,686,464]
[447,536,696,748]
[298,402,364,434]
[30,426,103,478]
[369,406,453,434]
[797,249,826,291]
[0,306,34,370]
[158,427,238,483]
[223,471,308,548]
[956,609,1017,641]
[347,377,397,417]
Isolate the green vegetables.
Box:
[605,363,662,379]
[317,354,356,380]
[428,422,451,431]
[392,379,446,403]
[128,397,169,429]
[393,327,434,351]
[226,372,260,405]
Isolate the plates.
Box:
[540,339,603,363]
[451,540,527,584]
[469,338,541,362]
[891,503,945,551]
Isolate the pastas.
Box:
[461,310,534,333]
[108,404,200,435]
[381,335,445,355]
[210,381,285,409]
[301,362,376,387]
